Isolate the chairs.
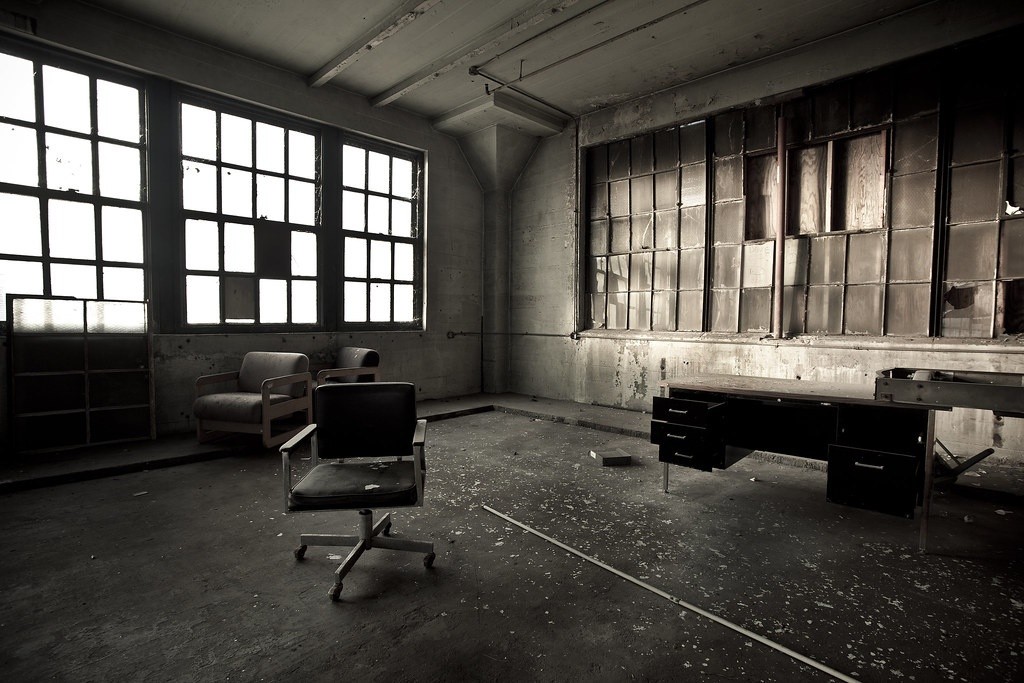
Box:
[279,381,436,601]
[935,439,995,494]
[192,351,313,449]
[312,347,380,393]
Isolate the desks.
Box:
[650,372,953,554]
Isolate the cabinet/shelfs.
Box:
[6,294,157,454]
